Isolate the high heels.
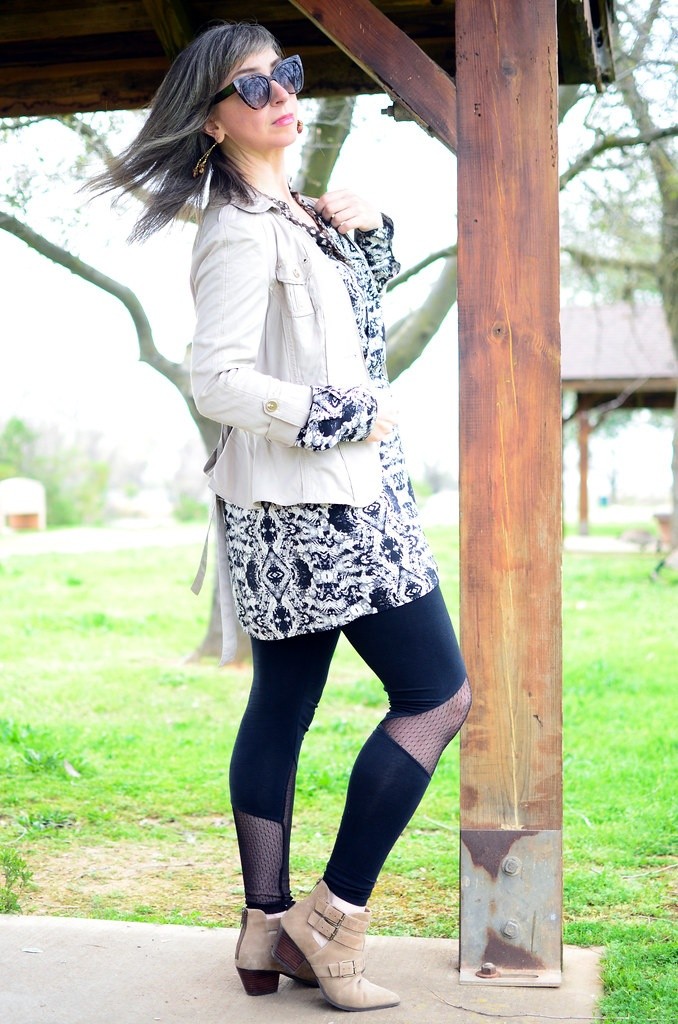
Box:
[270,878,400,1012]
[234,907,320,996]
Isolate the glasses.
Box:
[208,54,304,110]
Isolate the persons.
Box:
[79,23,471,1010]
